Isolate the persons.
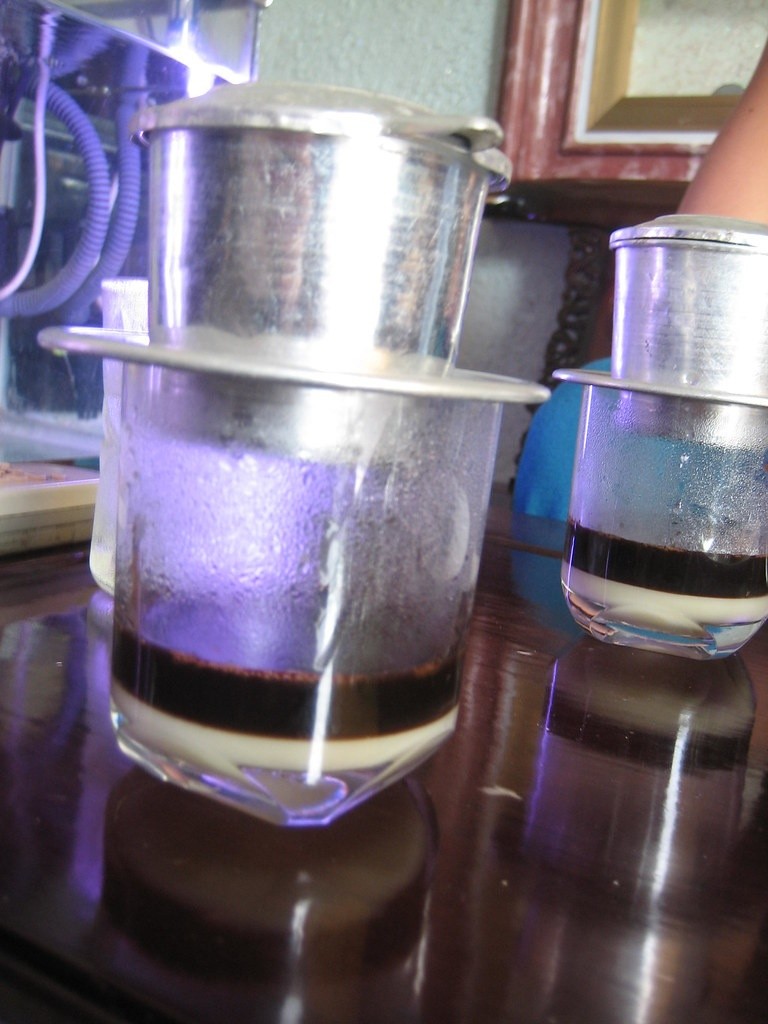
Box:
[514,42,768,523]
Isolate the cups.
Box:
[90,279,148,593]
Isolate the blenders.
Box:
[36,78,554,834]
[552,211,766,658]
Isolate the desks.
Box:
[0,531,768,1024]
